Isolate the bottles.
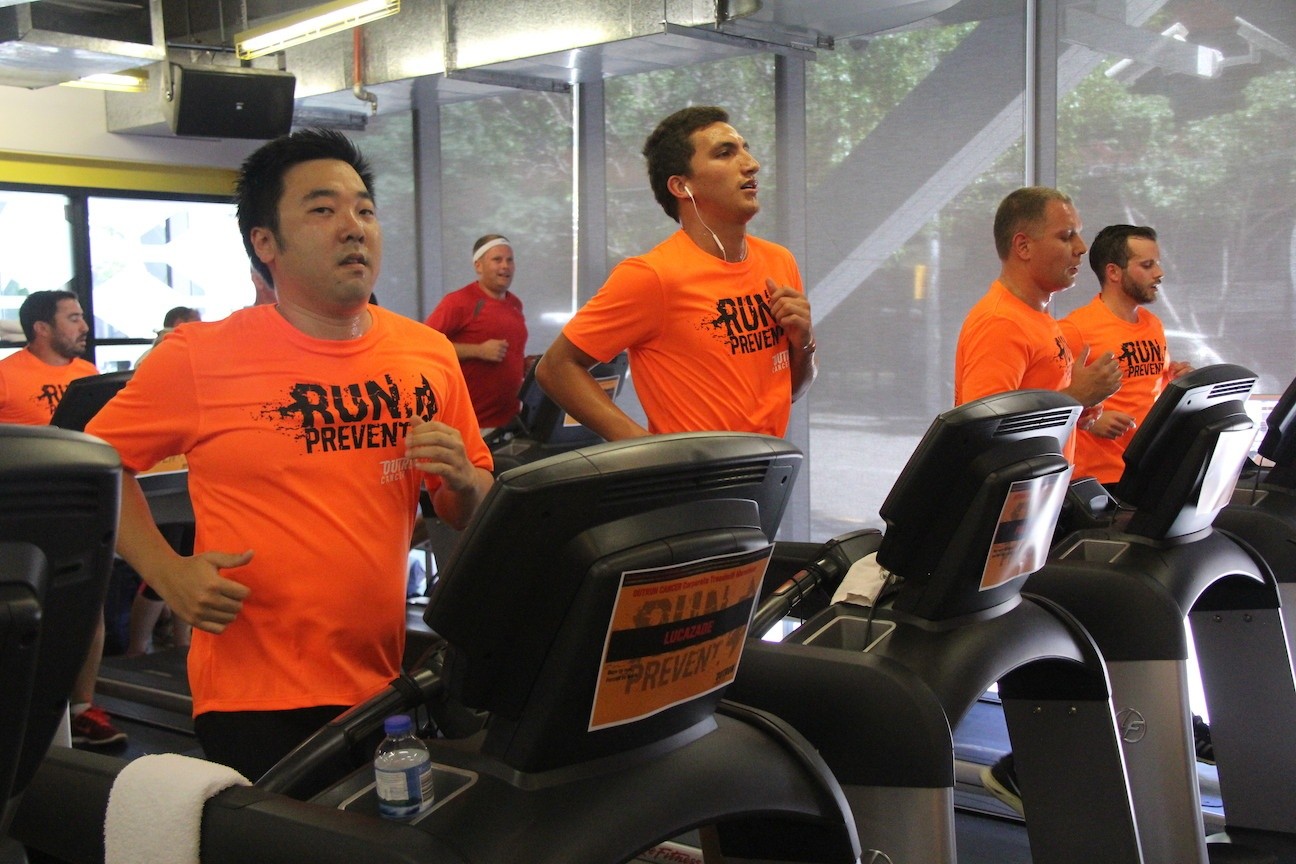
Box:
[371,716,436,825]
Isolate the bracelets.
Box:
[1081,412,1103,430]
[788,337,817,357]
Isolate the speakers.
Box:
[104,61,297,139]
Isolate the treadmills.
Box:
[0,350,1295,861]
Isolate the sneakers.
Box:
[72,705,130,745]
[980,754,1026,817]
[1191,715,1216,764]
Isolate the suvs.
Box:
[1152,328,1282,469]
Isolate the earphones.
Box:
[685,185,693,197]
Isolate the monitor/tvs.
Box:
[0,0,166,88]
[1,350,1295,805]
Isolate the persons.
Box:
[84,131,494,803]
[953,186,1219,816]
[536,107,817,446]
[0,234,523,750]
